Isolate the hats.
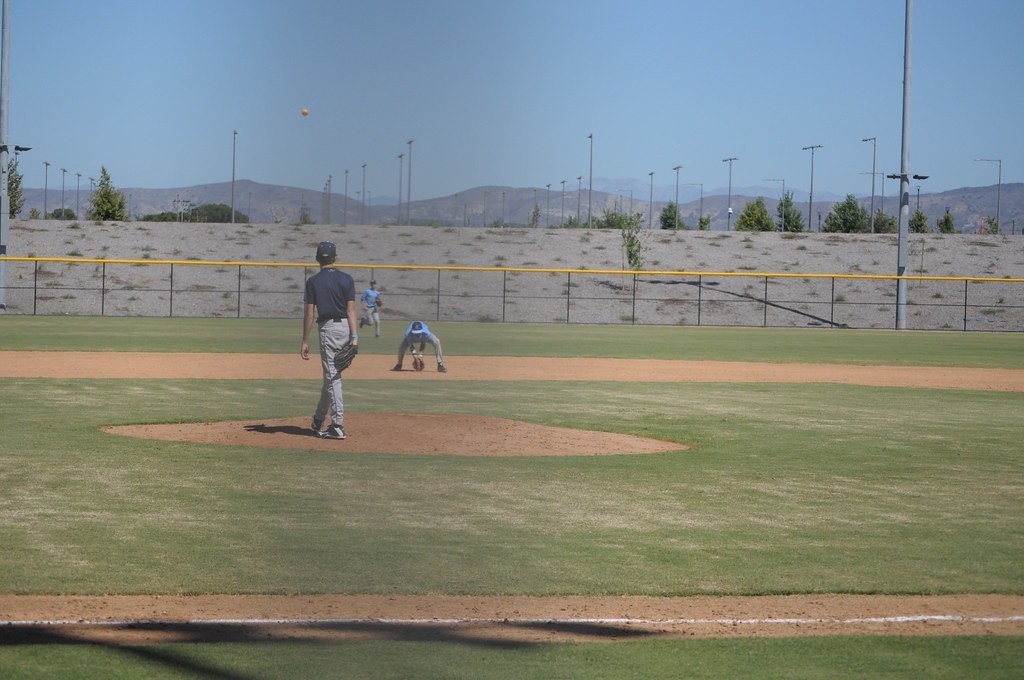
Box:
[411,322,423,333]
[370,280,376,285]
[317,241,336,260]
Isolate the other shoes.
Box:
[390,364,402,371]
[376,334,379,337]
[359,318,365,327]
[438,365,445,372]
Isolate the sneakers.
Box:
[311,419,322,433]
[315,424,346,439]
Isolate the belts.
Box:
[333,318,341,322]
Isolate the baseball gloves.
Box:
[412,357,425,372]
[333,341,359,372]
[376,297,383,307]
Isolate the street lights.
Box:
[405,139,416,226]
[560,180,567,228]
[60,168,68,220]
[802,145,824,233]
[321,175,333,224]
[42,160,51,220]
[588,133,593,228]
[231,129,239,223]
[648,172,657,229]
[722,157,740,231]
[88,177,95,220]
[344,170,349,223]
[862,137,876,233]
[546,184,551,228]
[397,153,406,226]
[973,159,1002,234]
[577,177,582,227]
[13,145,33,220]
[75,173,82,220]
[672,166,683,230]
[361,164,367,225]
[762,179,785,232]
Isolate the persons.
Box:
[301,240,358,439]
[391,320,448,372]
[359,280,383,337]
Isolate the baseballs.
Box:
[300,108,311,116]
